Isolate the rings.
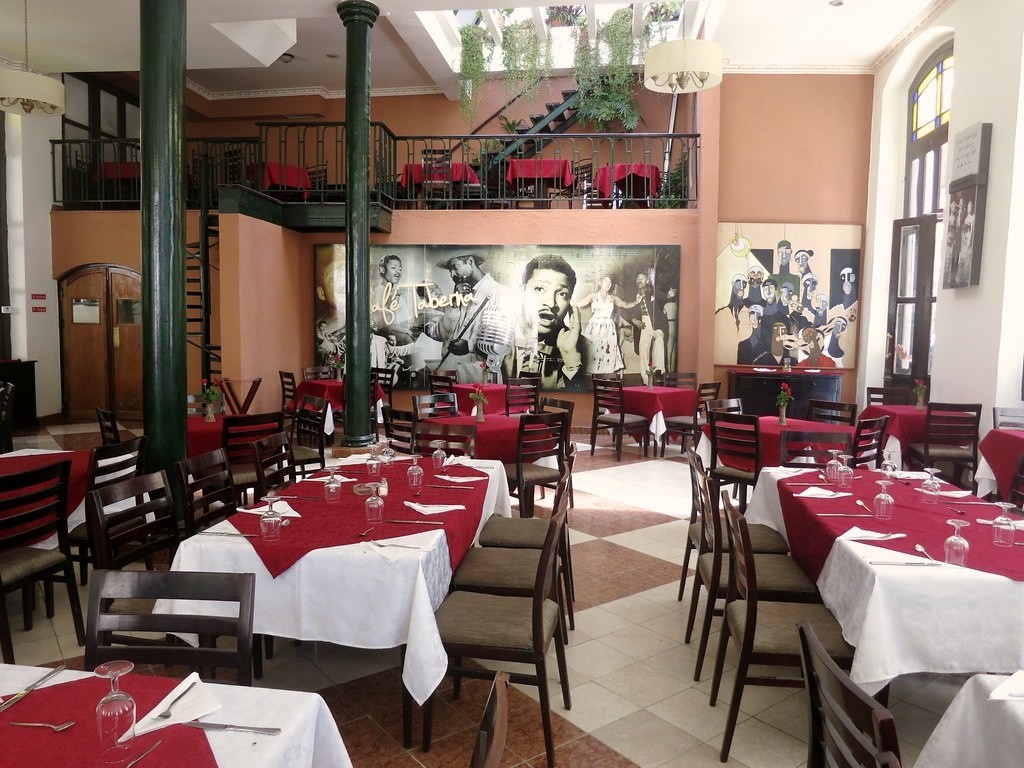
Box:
[464,286,466,290]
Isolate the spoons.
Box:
[359,527,374,536]
[10,721,75,732]
[899,480,911,484]
[414,490,422,495]
[948,507,965,514]
[819,474,938,563]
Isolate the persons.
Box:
[943,198,974,283]
[317,249,677,391]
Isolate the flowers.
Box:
[546,5,583,24]
[194,377,225,404]
[776,383,794,406]
[648,362,657,376]
[470,381,488,406]
[913,379,925,396]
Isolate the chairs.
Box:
[909,403,984,498]
[72,148,607,210]
[993,406,1024,431]
[711,489,894,763]
[403,491,570,766]
[0,374,910,688]
[796,621,904,768]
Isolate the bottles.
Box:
[378,478,388,497]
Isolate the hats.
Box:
[434,247,488,269]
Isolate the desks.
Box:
[729,370,844,424]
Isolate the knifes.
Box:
[817,467,828,476]
[785,480,941,565]
[185,722,281,735]
[449,465,494,469]
[904,478,1024,545]
[320,470,365,474]
[386,520,443,524]
[272,496,321,500]
[198,532,259,536]
[426,485,474,490]
[0,665,66,713]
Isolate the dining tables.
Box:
[439,382,535,425]
[610,385,698,456]
[149,412,1023,767]
[290,381,386,443]
[88,157,656,209]
[3,449,141,557]
[0,665,353,767]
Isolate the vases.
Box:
[476,403,485,423]
[917,394,924,411]
[551,18,562,26]
[777,405,787,426]
[204,402,215,421]
[647,376,654,389]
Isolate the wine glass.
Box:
[365,483,384,523]
[94,661,136,764]
[260,438,395,541]
[407,440,446,486]
[827,449,970,566]
[990,500,1016,548]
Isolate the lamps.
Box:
[280,53,294,64]
[645,0,722,94]
[0,0,65,115]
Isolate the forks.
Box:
[371,540,421,549]
[151,682,196,720]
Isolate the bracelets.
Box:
[566,364,582,371]
